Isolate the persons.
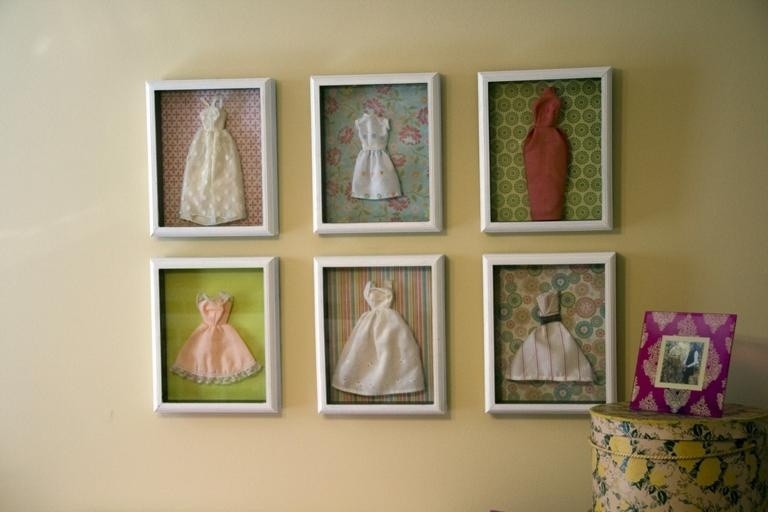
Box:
[679,343,698,383]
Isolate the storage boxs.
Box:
[587,399,768,512]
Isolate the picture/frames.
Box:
[149,256,282,415]
[629,310,737,418]
[144,77,278,238]
[313,254,448,418]
[482,253,616,416]
[477,67,614,234]
[310,73,442,237]
